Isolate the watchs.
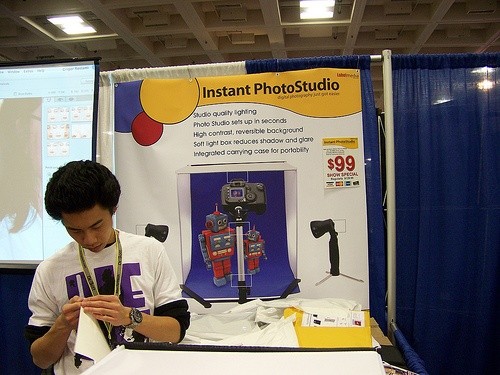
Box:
[122,307,143,329]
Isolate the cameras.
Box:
[221,178,267,215]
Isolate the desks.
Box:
[77,344,386,375]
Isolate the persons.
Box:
[25,159,191,375]
[1,97,43,261]
